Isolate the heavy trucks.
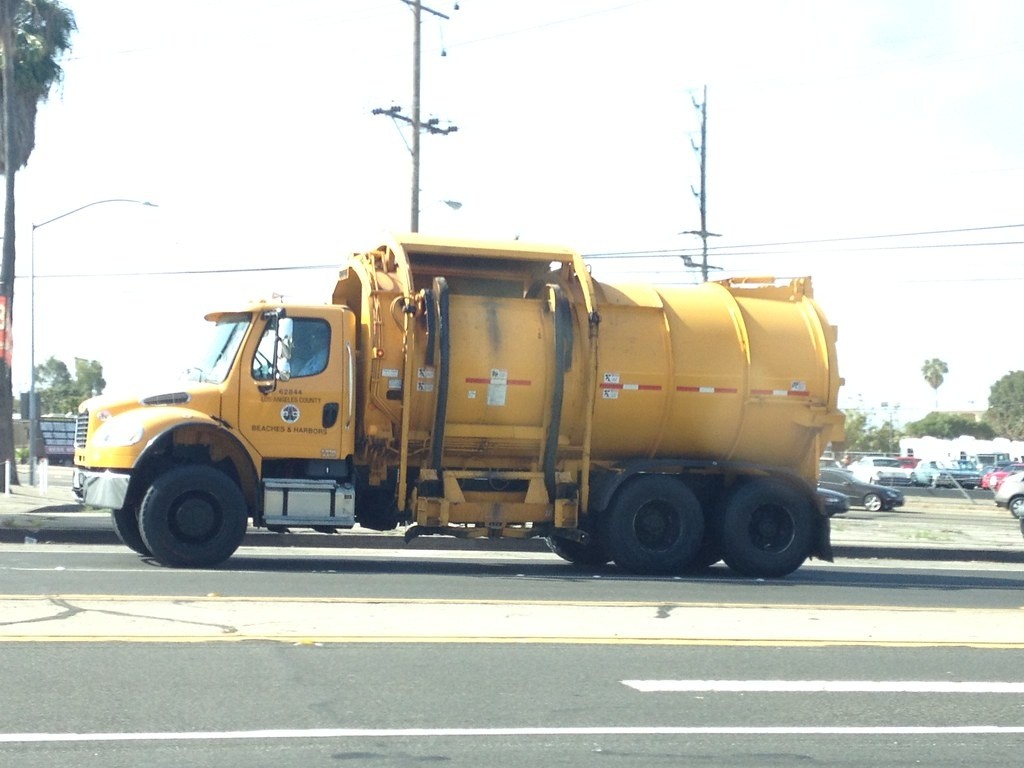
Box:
[71,233,846,578]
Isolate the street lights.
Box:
[29,198,160,486]
[411,199,462,232]
[882,402,900,457]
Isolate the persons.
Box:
[289,324,329,379]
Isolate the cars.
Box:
[819,458,843,470]
[819,469,905,512]
[914,459,982,489]
[897,457,918,470]
[995,471,1024,519]
[952,460,1024,491]
[850,456,913,487]
[818,488,850,517]
